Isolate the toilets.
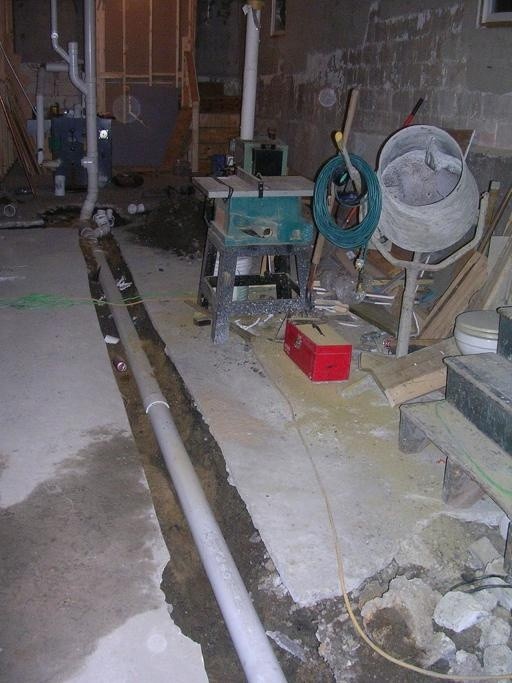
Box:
[453,310,500,355]
